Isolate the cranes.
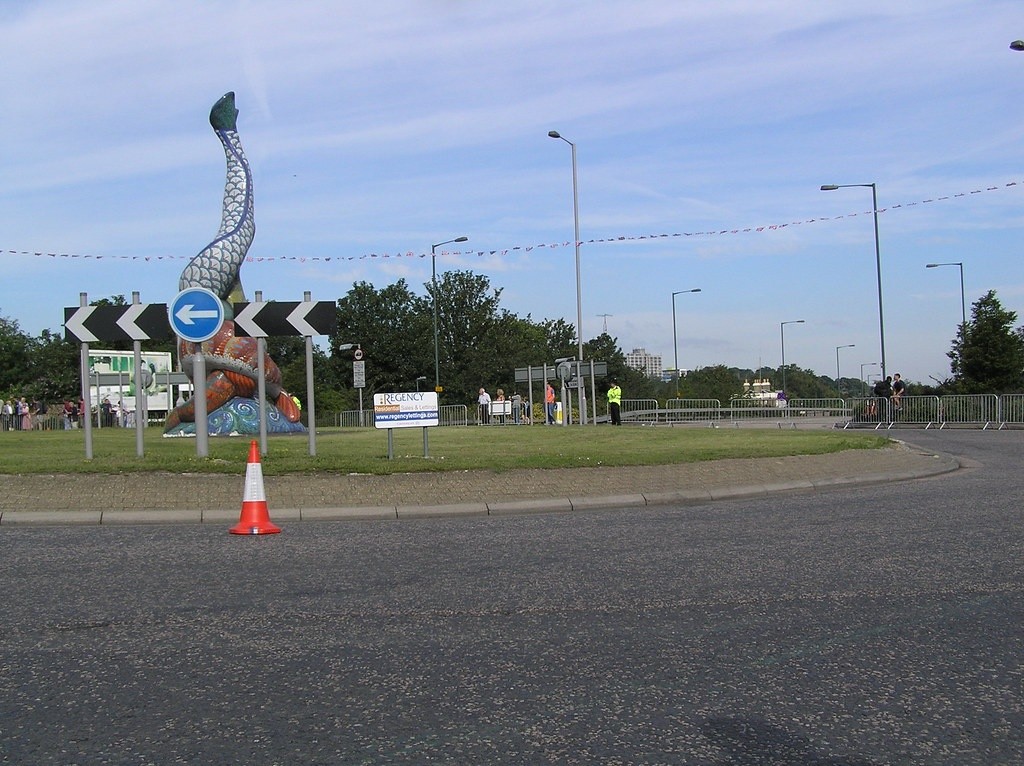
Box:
[596,313,613,333]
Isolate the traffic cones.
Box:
[229,439,281,536]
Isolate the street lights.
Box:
[549,130,587,425]
[861,363,876,397]
[672,288,702,421]
[432,236,469,403]
[781,320,806,393]
[837,344,855,408]
[819,183,886,382]
[868,374,881,386]
[416,376,427,392]
[926,262,965,335]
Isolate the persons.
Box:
[891,373,904,411]
[879,376,894,425]
[543,382,556,425]
[866,395,877,423]
[478,388,491,424]
[497,389,505,424]
[522,396,530,424]
[607,378,621,426]
[509,390,521,423]
[0,398,136,431]
